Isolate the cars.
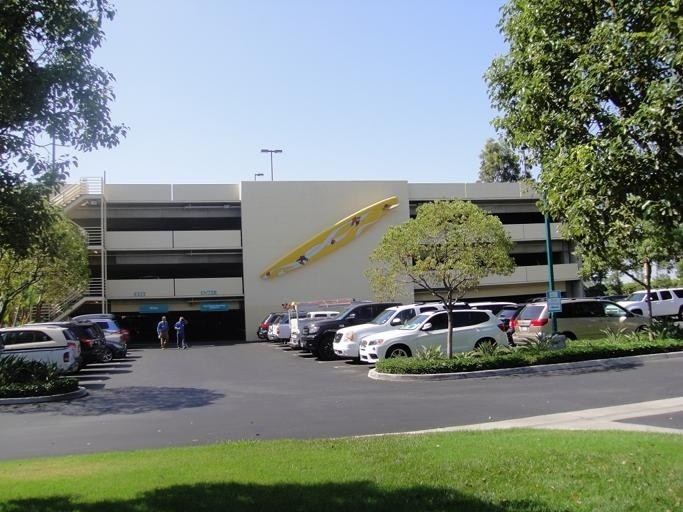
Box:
[0,313,129,376]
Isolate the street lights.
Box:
[261,148,282,181]
[254,173,263,180]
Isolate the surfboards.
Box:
[259,195,398,280]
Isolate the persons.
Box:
[155,315,169,351]
[173,315,189,350]
